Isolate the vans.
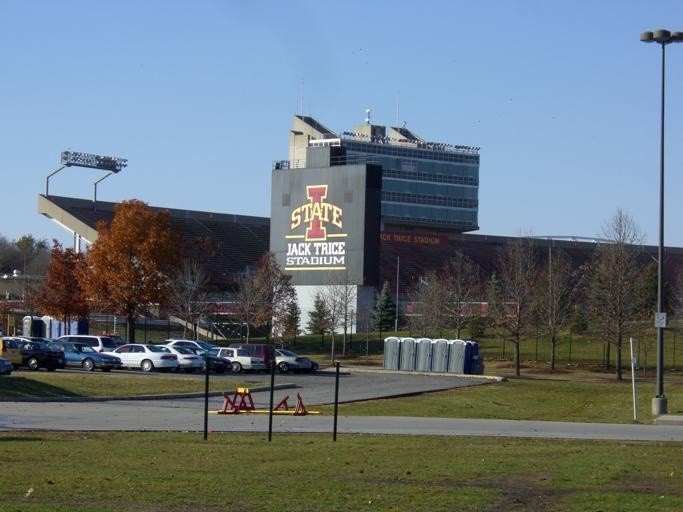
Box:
[229,343,274,374]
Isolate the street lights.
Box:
[639,30,682,396]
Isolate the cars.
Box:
[0,335,267,375]
[274,349,319,374]
